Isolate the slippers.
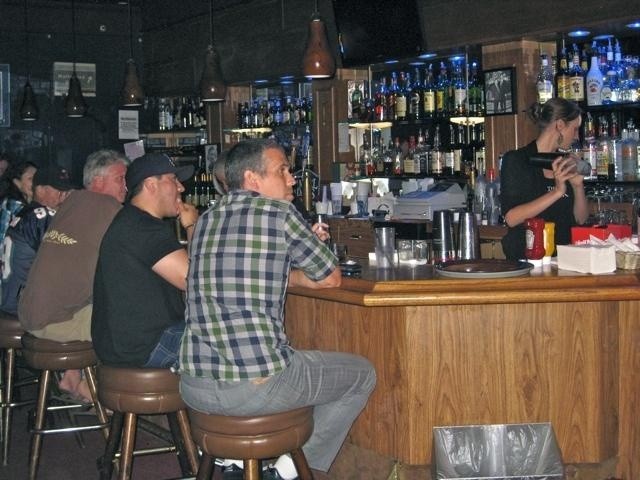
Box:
[50,388,114,417]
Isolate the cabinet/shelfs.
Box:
[140,20,640,210]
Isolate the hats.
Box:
[125,152,194,189]
[32,163,82,192]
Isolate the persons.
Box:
[17,148,131,419]
[0,160,40,241]
[0,153,13,198]
[0,164,83,316]
[500,98,590,257]
[176,138,377,479]
[485,71,510,113]
[213,151,309,220]
[90,151,200,371]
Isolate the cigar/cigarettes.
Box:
[318,214,322,230]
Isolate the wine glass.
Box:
[586,184,639,226]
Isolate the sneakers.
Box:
[221,458,301,479]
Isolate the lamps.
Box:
[119,0,144,107]
[20,0,38,121]
[199,0,226,103]
[303,0,336,79]
[65,0,86,118]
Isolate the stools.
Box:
[190,404,314,480]
[96,366,198,478]
[2,318,35,468]
[23,330,121,479]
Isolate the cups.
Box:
[374,227,430,269]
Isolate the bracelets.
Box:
[184,223,194,231]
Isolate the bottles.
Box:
[238,94,313,167]
[157,97,217,208]
[538,37,640,182]
[314,201,331,247]
[523,217,555,264]
[352,61,503,225]
[432,210,480,266]
